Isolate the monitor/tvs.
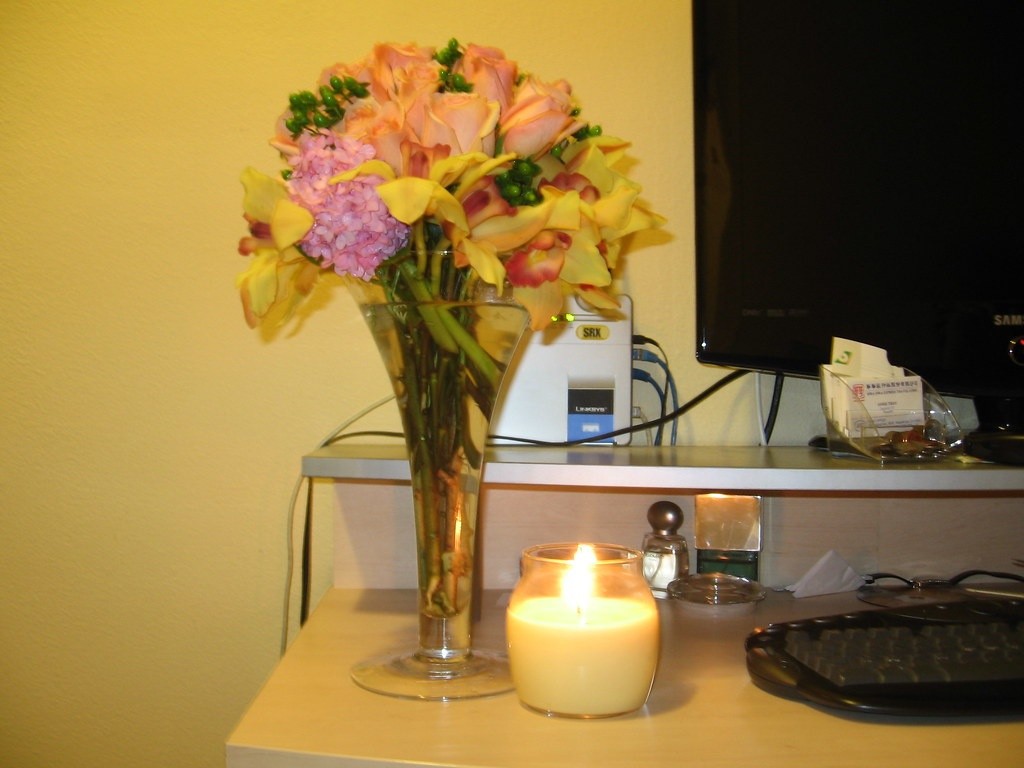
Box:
[694,0,1024,461]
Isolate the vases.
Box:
[339,252,518,698]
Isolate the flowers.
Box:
[238,39,671,613]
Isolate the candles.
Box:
[507,542,660,717]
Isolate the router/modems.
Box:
[484,293,634,447]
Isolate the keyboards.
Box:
[746,598,1024,717]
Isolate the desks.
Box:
[225,447,1024,768]
